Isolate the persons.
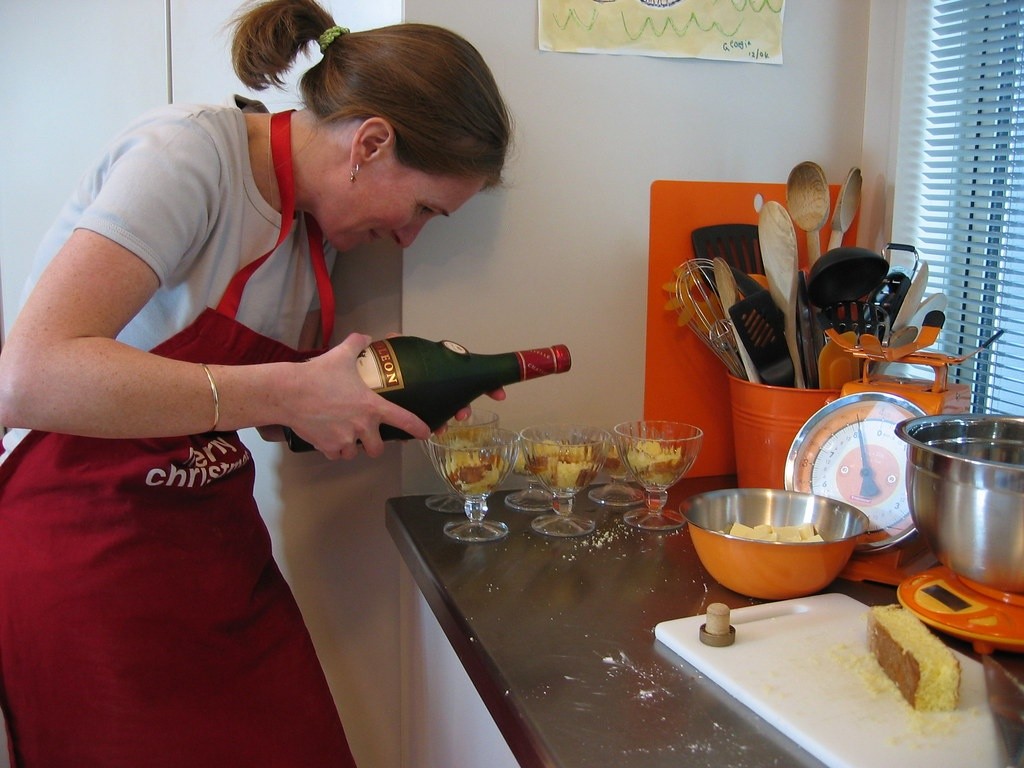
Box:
[1,0,509,768]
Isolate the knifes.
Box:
[982,654,1024,768]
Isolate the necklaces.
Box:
[269,113,274,207]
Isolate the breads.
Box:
[865,602,961,713]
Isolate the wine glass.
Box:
[521,423,614,537]
[428,427,521,541]
[614,420,703,530]
[576,428,645,506]
[502,433,554,511]
[419,408,499,512]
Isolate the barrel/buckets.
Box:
[726,369,842,491]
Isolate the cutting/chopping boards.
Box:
[641,179,860,479]
[654,592,1024,768]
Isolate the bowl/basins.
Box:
[679,488,869,600]
[895,414,1024,593]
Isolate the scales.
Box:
[781,308,1007,586]
[895,564,1024,658]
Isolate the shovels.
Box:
[818,297,890,378]
[690,221,765,295]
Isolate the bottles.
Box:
[282,336,573,451]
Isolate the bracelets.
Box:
[200,363,219,429]
